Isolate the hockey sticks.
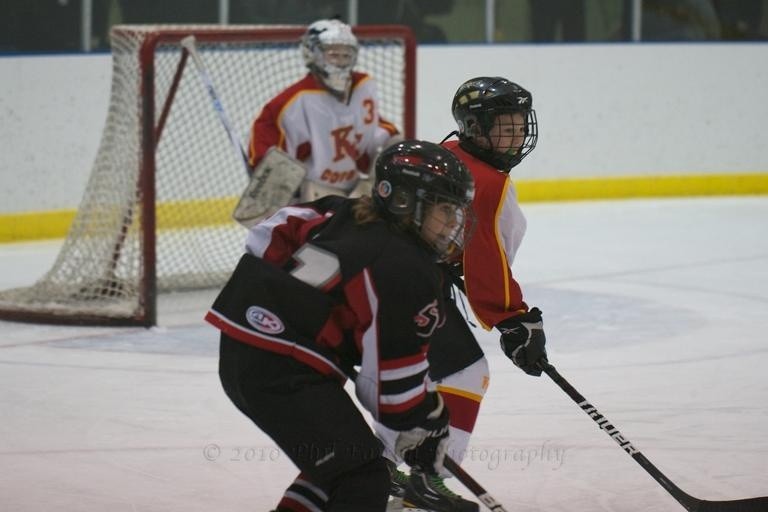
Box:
[442,263,768,511]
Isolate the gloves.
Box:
[496,307,548,376]
[393,392,450,473]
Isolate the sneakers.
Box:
[390,467,479,512]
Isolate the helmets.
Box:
[452,76,538,174]
[373,140,477,264]
[300,18,359,97]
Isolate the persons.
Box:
[248,19,400,205]
[373,77,547,512]
[205,139,476,512]
